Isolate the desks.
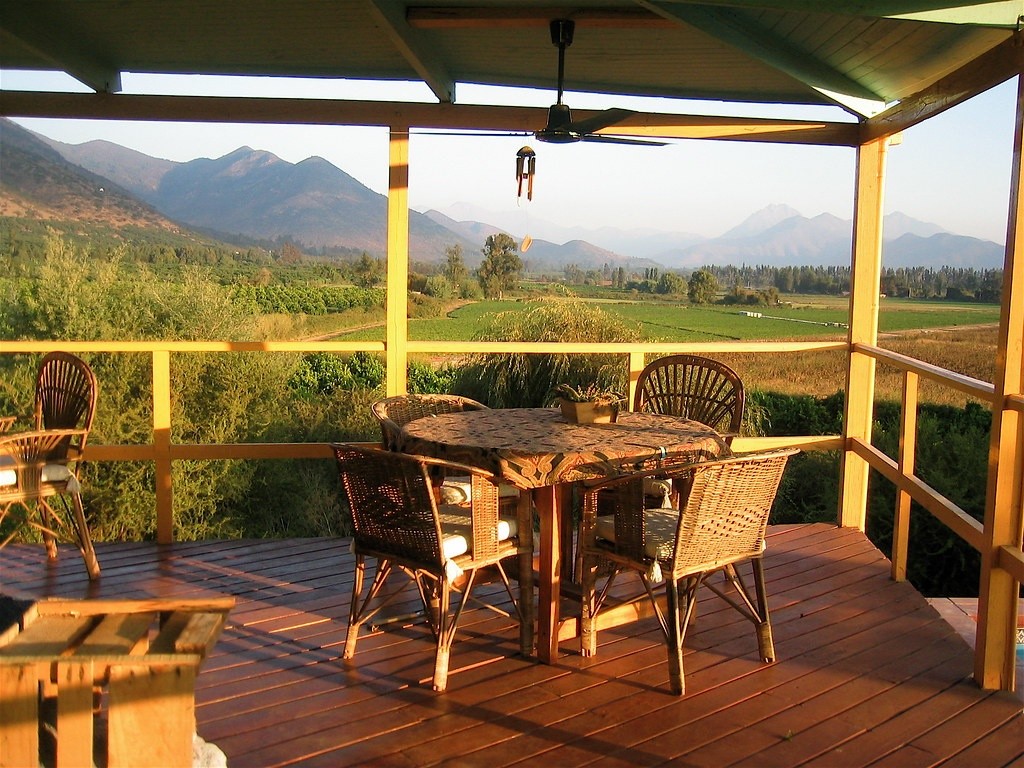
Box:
[388,408,734,665]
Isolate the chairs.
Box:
[575,354,746,583]
[370,394,518,587]
[0,350,103,583]
[580,447,799,695]
[332,442,536,692]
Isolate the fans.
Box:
[388,20,675,149]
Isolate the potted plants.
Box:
[541,383,628,423]
